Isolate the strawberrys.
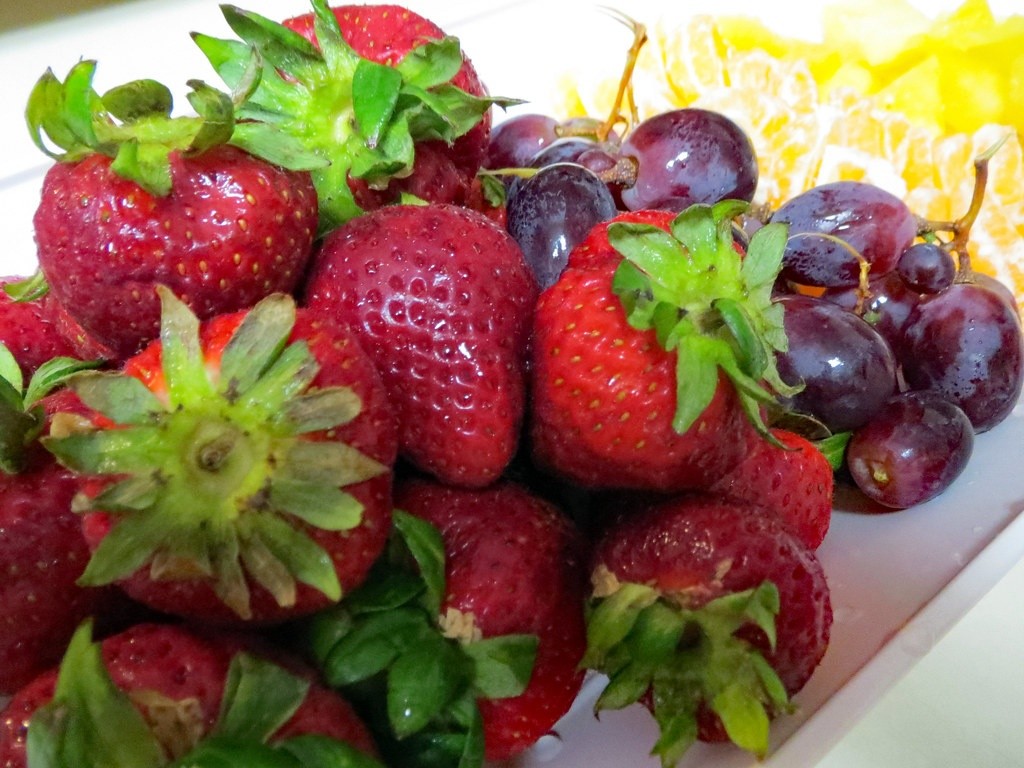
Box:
[1,7,835,767]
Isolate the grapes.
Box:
[490,20,1024,510]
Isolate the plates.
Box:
[0,1,1023,765]
